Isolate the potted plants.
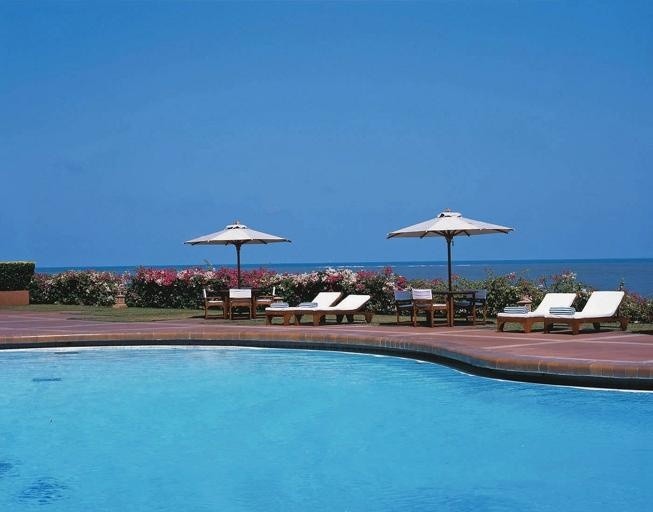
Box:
[0,261,35,306]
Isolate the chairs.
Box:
[495,290,628,335]
[202,284,487,327]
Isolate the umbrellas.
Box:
[185,220,291,289]
[387,207,514,292]
